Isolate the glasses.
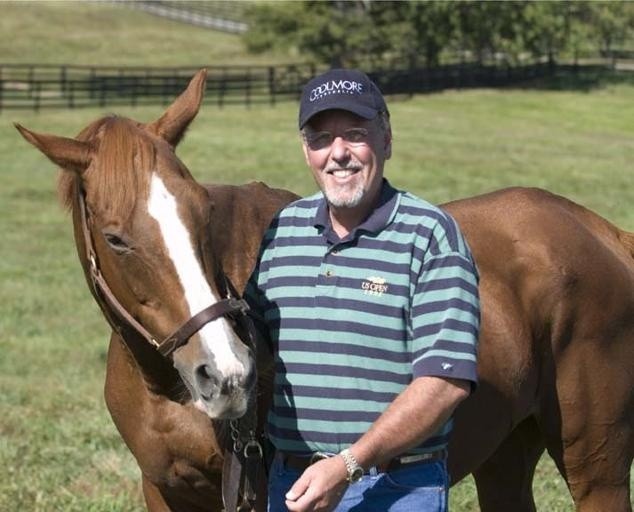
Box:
[302,127,382,146]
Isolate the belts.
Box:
[275,449,444,474]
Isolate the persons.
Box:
[242,68,480,512]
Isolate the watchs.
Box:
[340,450,365,484]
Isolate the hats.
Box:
[299,69,390,130]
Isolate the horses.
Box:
[14,70,634,512]
